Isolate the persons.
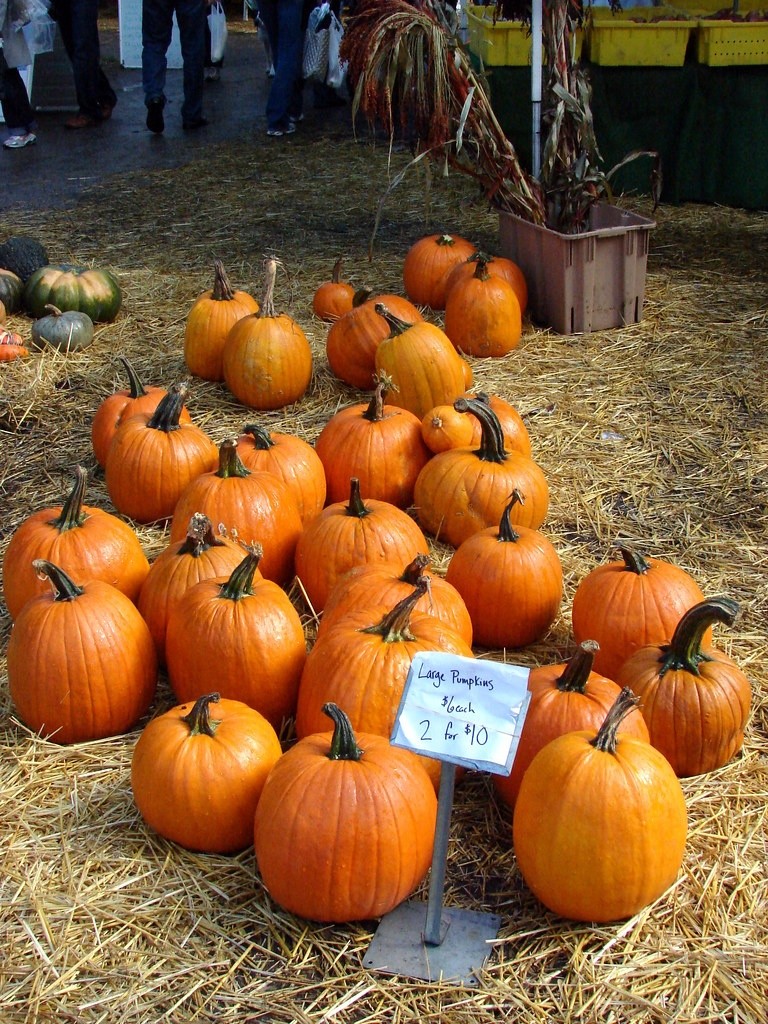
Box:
[242,0,349,136]
[0,0,118,148]
[140,0,212,133]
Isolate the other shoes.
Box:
[289,112,304,123]
[265,64,276,78]
[146,98,165,133]
[203,66,220,82]
[182,113,209,127]
[266,123,295,135]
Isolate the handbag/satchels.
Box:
[325,6,351,101]
[300,3,331,82]
[206,1,228,63]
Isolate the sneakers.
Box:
[3,129,36,148]
[66,115,95,127]
[98,101,115,121]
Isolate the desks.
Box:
[463,44,768,212]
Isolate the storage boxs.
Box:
[487,200,659,337]
[583,6,698,69]
[463,6,589,70]
[687,8,768,68]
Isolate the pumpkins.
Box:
[93,257,563,650]
[252,704,439,920]
[625,7,768,23]
[294,583,479,791]
[3,467,150,621]
[132,693,282,855]
[404,231,528,360]
[8,558,156,743]
[167,545,306,730]
[488,543,749,925]
[0,235,121,363]
